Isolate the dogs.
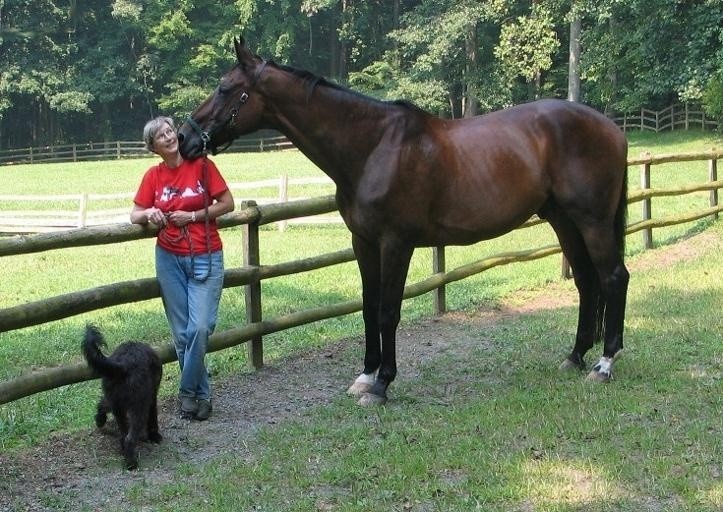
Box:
[81,324,162,471]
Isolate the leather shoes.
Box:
[194,398,212,420]
[176,391,198,413]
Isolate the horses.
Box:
[177,33,630,408]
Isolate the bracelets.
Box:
[191,212,196,222]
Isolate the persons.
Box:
[127,114,235,422]
